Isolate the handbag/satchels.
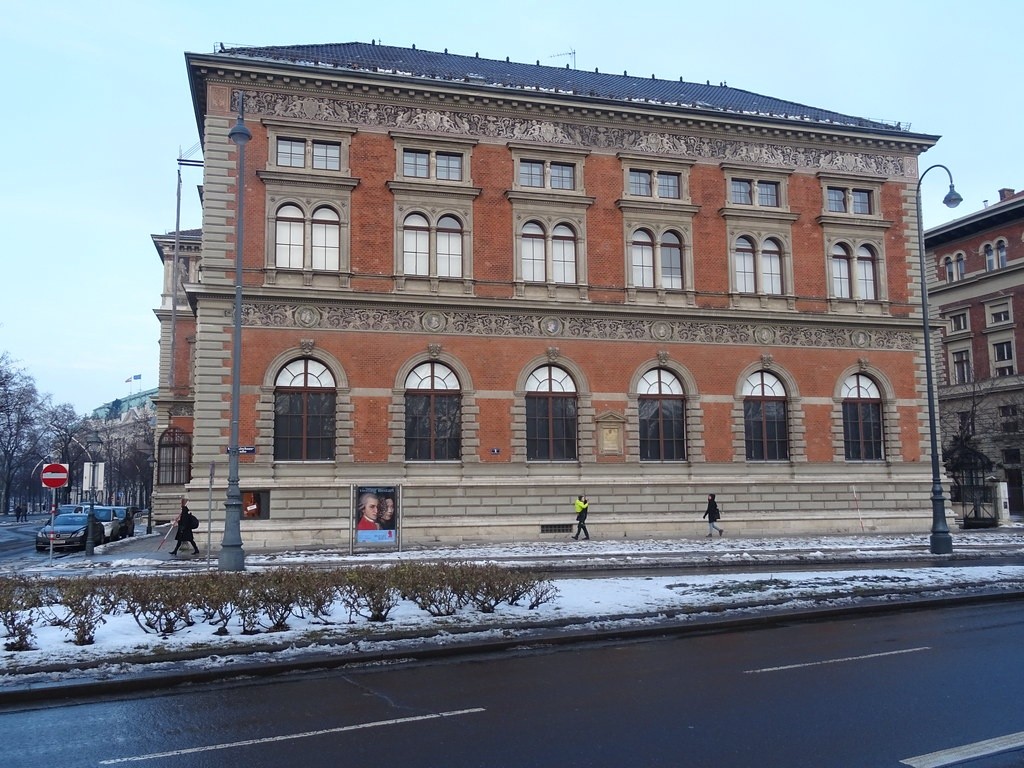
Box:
[715,508,720,519]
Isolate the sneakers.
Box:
[718,528,723,537]
[705,534,712,537]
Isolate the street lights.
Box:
[217,95,253,572]
[918,161,967,557]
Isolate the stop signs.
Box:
[41,464,70,487]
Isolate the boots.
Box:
[190,547,199,555]
[169,546,178,555]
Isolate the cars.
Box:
[111,506,135,539]
[46,501,101,523]
[83,507,119,542]
[36,513,105,553]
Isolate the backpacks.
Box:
[184,514,199,528]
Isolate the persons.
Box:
[575,495,589,526]
[572,500,590,540]
[21,505,27,522]
[168,506,200,555]
[357,493,382,529]
[376,496,394,529]
[16,507,21,522]
[703,495,724,537]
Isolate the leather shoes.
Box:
[581,536,590,540]
[572,534,579,540]
